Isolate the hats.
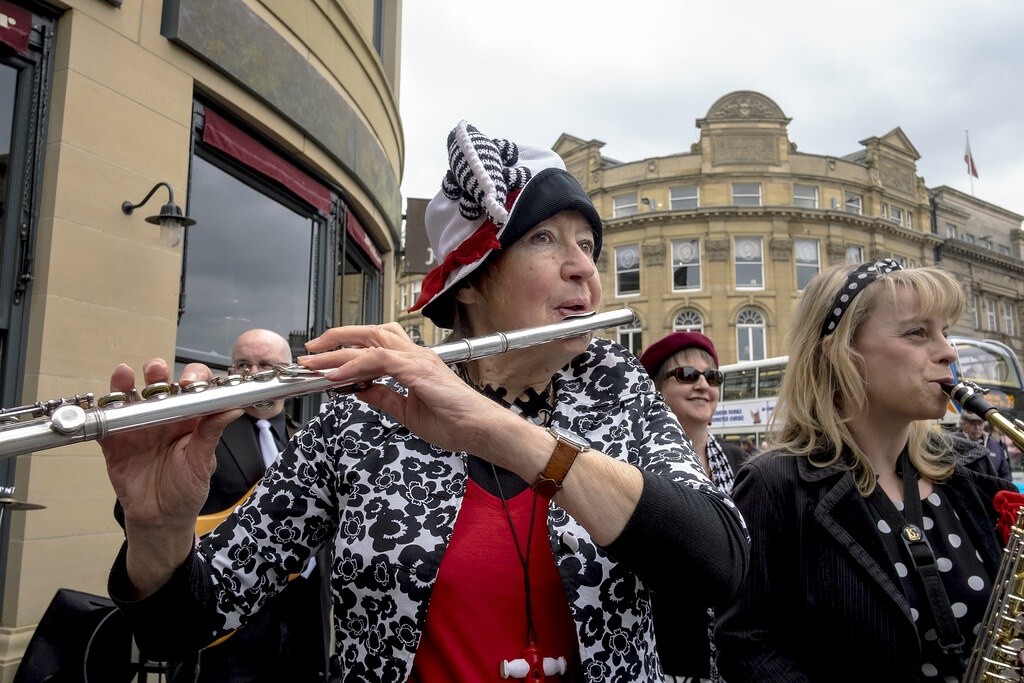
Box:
[961,409,984,421]
[638,331,721,381]
[408,119,603,328]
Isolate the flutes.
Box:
[0,305,635,463]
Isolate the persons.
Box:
[197,328,306,517]
[637,332,754,497]
[718,260,1023,683]
[100,122,751,683]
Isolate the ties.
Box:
[256,419,316,578]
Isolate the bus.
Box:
[706,334,1024,496]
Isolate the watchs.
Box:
[531,426,591,498]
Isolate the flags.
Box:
[964,134,979,179]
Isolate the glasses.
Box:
[659,366,724,386]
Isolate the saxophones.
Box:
[940,381,1024,683]
